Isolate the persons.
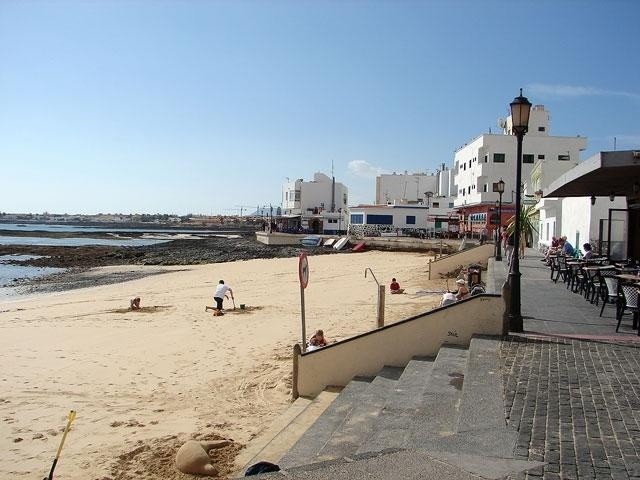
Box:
[455,279,469,300]
[390,278,405,294]
[128,297,141,308]
[309,329,325,346]
[540,236,593,266]
[498,225,526,266]
[206,280,234,311]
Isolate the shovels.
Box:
[42,409,76,480]
[233,298,236,310]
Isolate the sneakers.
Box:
[540,258,551,266]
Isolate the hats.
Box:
[456,279,464,284]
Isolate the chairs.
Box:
[548,247,639,334]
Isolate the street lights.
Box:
[510,88,532,333]
[495,177,505,260]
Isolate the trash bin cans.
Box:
[468,264,481,295]
